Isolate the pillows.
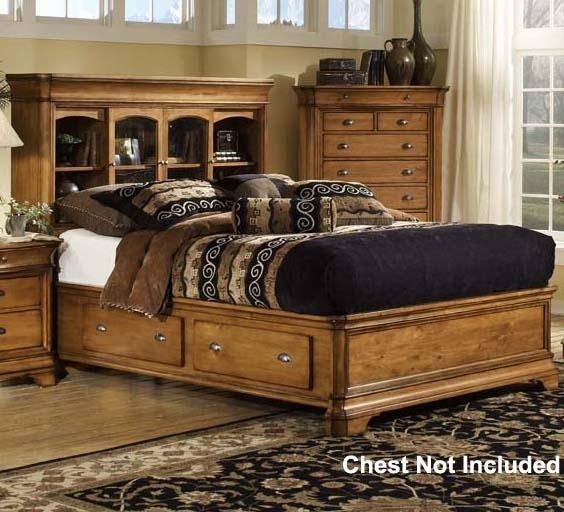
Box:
[53,180,143,238]
[209,173,296,201]
[89,177,238,231]
[281,178,395,227]
[232,197,337,234]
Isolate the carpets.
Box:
[0,362,564,512]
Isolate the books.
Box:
[361,50,385,86]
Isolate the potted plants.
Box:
[0,193,52,237]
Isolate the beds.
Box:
[54,220,559,439]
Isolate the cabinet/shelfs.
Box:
[290,83,451,223]
[11,99,108,231]
[207,103,268,181]
[108,102,207,185]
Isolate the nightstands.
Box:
[0,233,63,388]
